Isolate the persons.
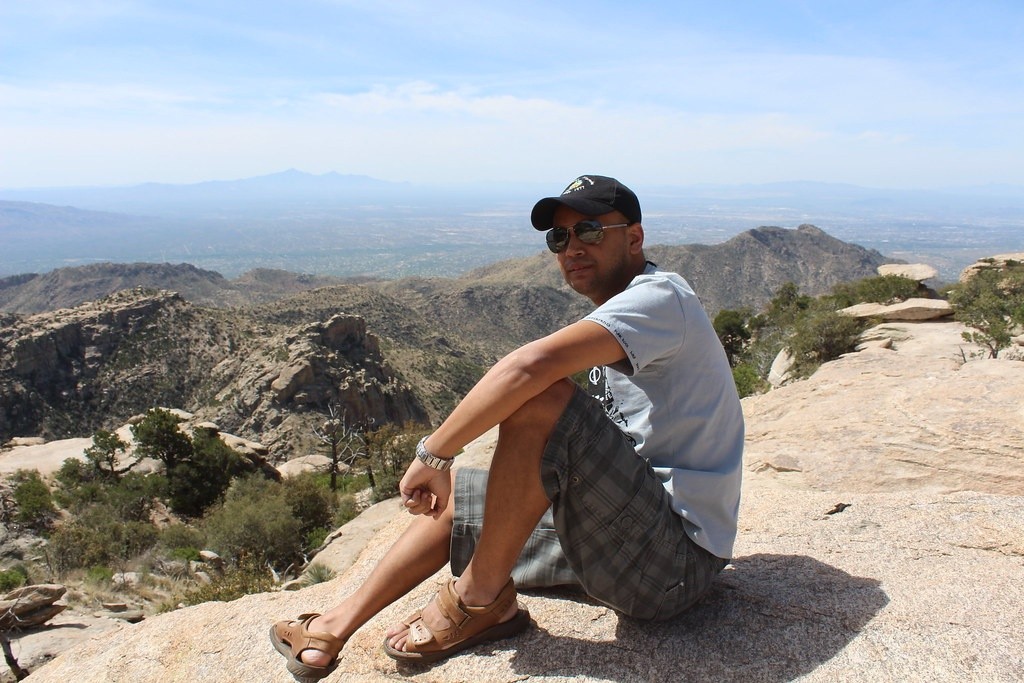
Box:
[266,176,745,683]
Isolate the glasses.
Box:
[545,219,629,254]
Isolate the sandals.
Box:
[382,576,531,664]
[269,612,346,677]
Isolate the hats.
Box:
[530,174,641,232]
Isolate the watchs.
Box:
[414,436,455,473]
